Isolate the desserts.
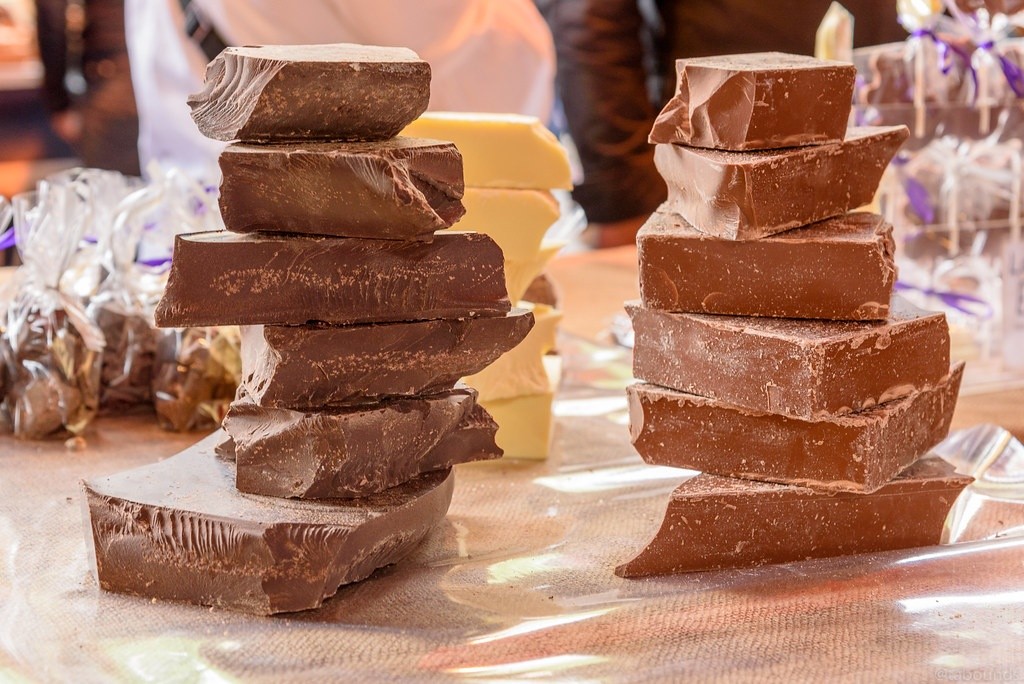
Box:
[82,45,572,615]
[613,53,975,579]
